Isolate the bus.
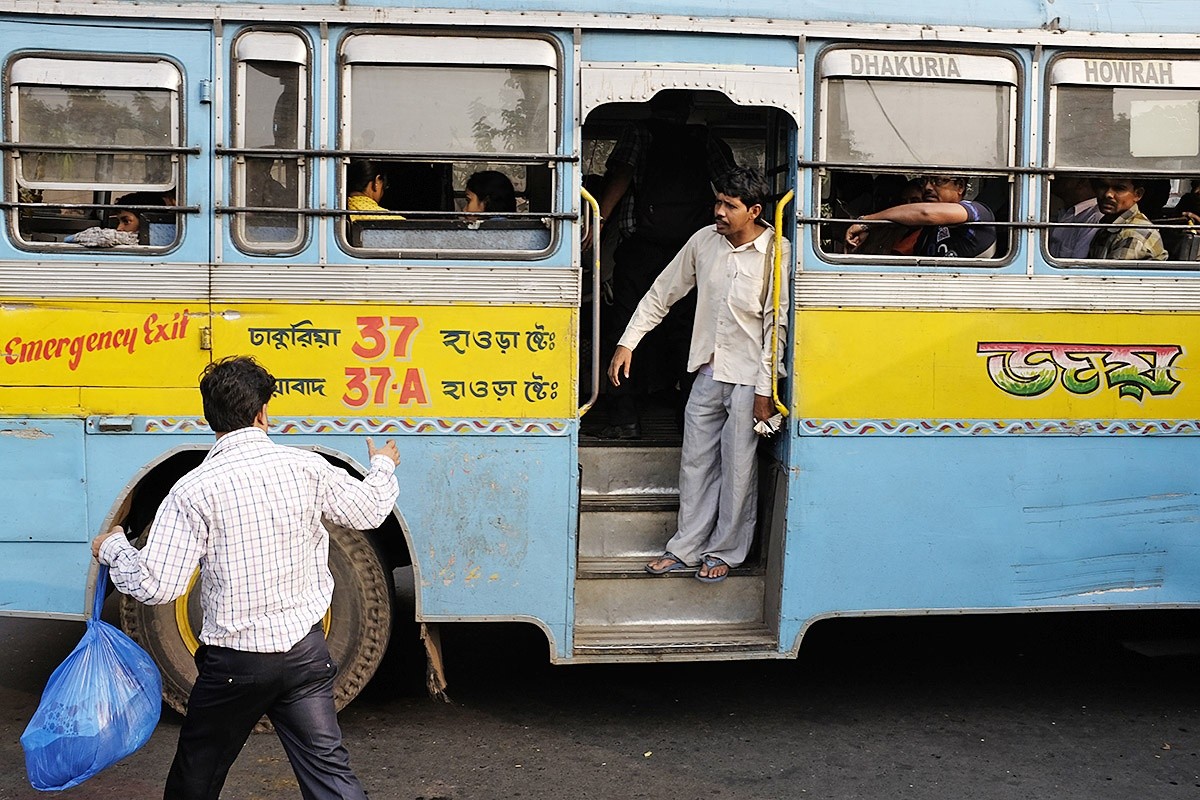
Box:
[0,1,1200,739]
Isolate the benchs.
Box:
[352,218,551,251]
[139,211,299,244]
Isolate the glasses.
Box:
[920,175,957,187]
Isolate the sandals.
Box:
[695,556,730,584]
[644,552,684,574]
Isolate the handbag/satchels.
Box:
[21,565,162,791]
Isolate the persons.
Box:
[607,165,792,581]
[60,78,1200,444]
[91,357,404,800]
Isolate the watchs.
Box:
[600,214,606,224]
[856,216,869,231]
